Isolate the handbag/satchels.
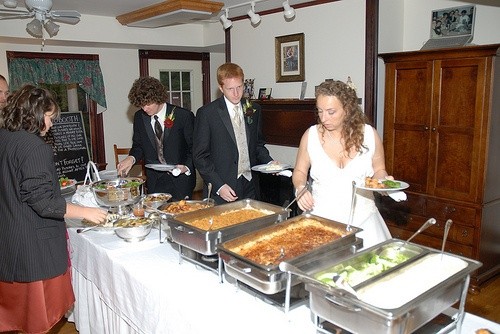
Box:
[71,161,101,207]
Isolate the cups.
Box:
[133,203,144,218]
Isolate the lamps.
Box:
[221,8,232,29]
[247,2,261,24]
[281,0,295,18]
[116,0,224,28]
[27,12,60,37]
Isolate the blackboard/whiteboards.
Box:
[40,111,93,184]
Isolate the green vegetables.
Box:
[59,177,71,187]
[94,181,141,189]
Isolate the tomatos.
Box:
[61,181,73,186]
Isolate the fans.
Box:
[0,0,81,49]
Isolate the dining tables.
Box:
[63,192,500,334]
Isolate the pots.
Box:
[157,198,215,218]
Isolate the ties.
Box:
[233,106,252,182]
[154,115,163,140]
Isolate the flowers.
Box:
[162,105,176,128]
[243,100,256,124]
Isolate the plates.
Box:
[144,164,177,171]
[355,180,409,191]
[252,164,291,173]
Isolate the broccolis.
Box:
[382,180,401,188]
[317,256,388,288]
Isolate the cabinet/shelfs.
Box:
[256,97,363,146]
[377,42,500,295]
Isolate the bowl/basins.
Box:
[142,193,172,209]
[89,177,145,206]
[114,217,154,241]
[59,179,77,201]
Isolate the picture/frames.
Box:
[274,32,305,82]
[258,87,272,99]
[430,4,476,38]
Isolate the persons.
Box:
[292,81,394,250]
[193,63,279,206]
[0,75,9,114]
[0,85,106,333]
[118,77,196,204]
[286,48,295,70]
[432,7,474,38]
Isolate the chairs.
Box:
[114,144,145,177]
